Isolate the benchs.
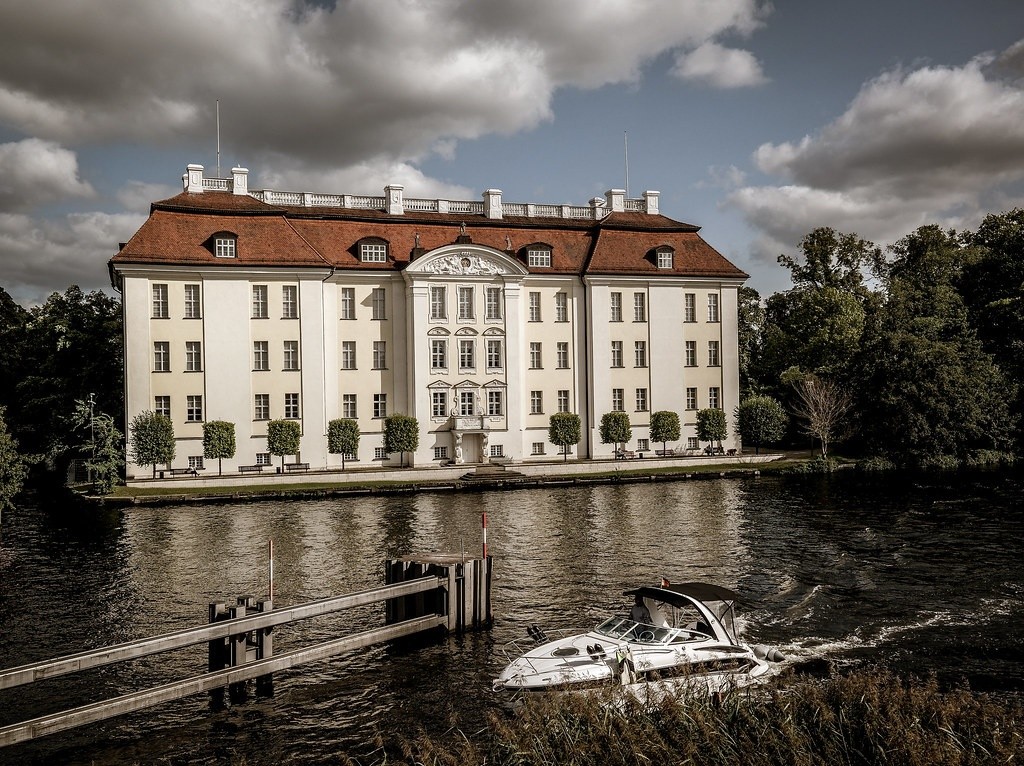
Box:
[705,447,726,456]
[615,450,637,459]
[285,463,310,473]
[655,449,675,457]
[238,466,263,475]
[169,469,196,478]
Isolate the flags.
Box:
[662,578,669,587]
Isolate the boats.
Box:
[492,577,788,718]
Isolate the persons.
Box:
[622,595,651,638]
